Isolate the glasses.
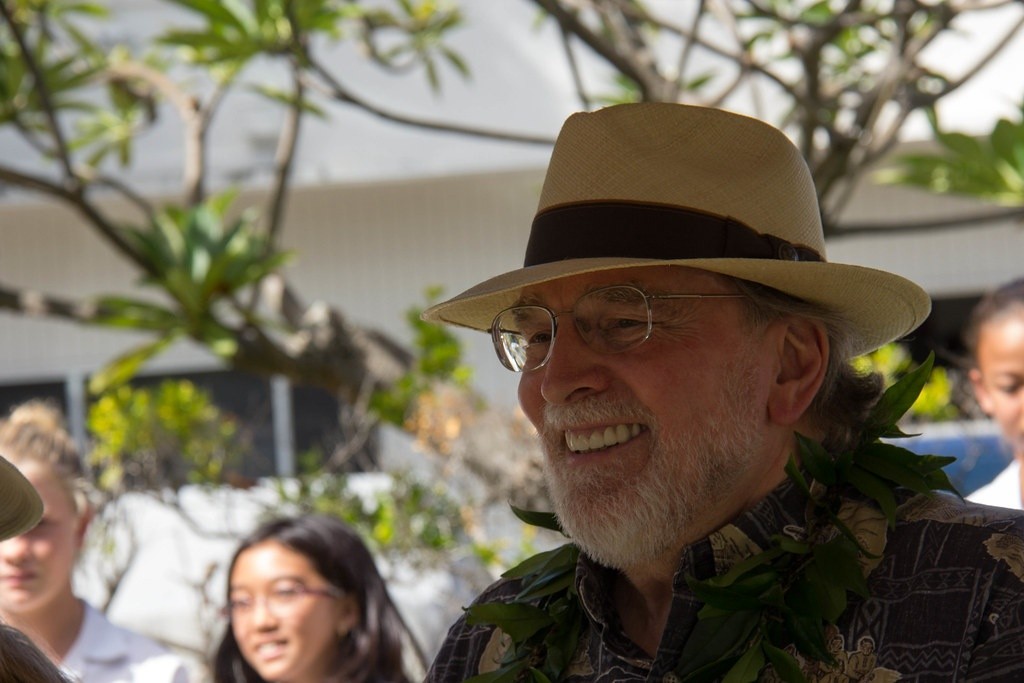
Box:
[222,576,350,618]
[487,284,746,373]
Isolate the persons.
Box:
[420,101,1024,683]
[0,400,210,683]
[212,512,427,683]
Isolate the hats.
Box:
[418,101,932,362]
[0,456,44,542]
